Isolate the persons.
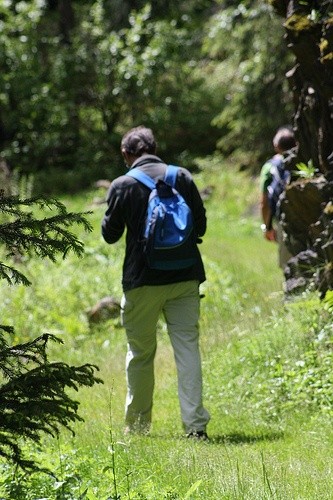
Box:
[101,126,209,440]
[260,128,329,297]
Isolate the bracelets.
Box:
[261,223,273,233]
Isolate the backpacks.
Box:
[125,162,193,255]
[265,156,292,211]
